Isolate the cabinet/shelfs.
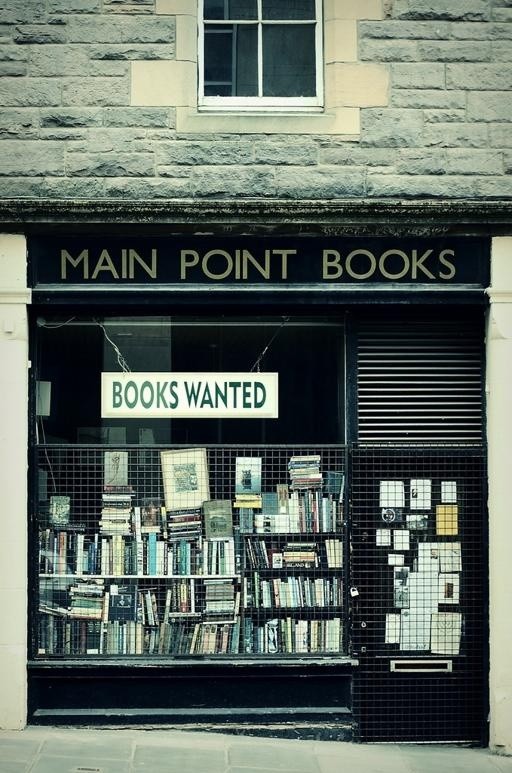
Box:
[29,444,349,661]
